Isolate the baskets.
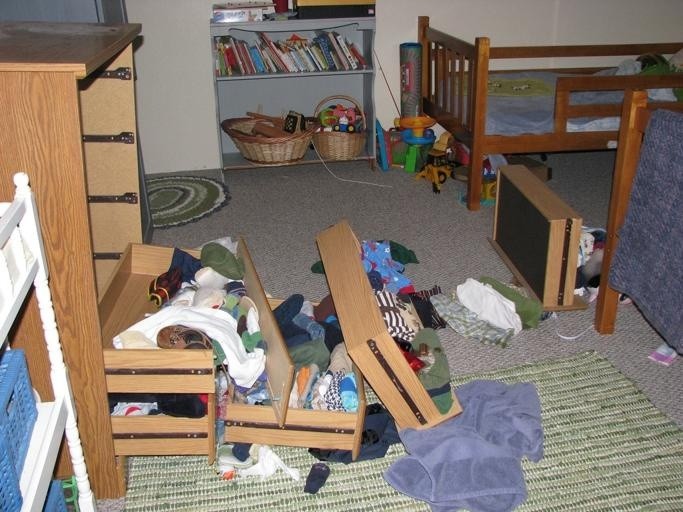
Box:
[220,112,321,163]
[312,96,367,160]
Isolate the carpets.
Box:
[117,350,683,512]
[145,171,232,230]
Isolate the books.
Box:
[214,29,370,74]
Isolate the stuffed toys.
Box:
[314,102,365,133]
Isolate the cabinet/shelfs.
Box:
[0,16,145,498]
[0,171,96,512]
[314,215,462,434]
[210,10,381,183]
[98,240,222,395]
[226,294,371,460]
[484,163,585,309]
[107,389,216,466]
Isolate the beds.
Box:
[415,14,683,211]
[593,82,683,360]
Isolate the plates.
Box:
[210,9,249,22]
